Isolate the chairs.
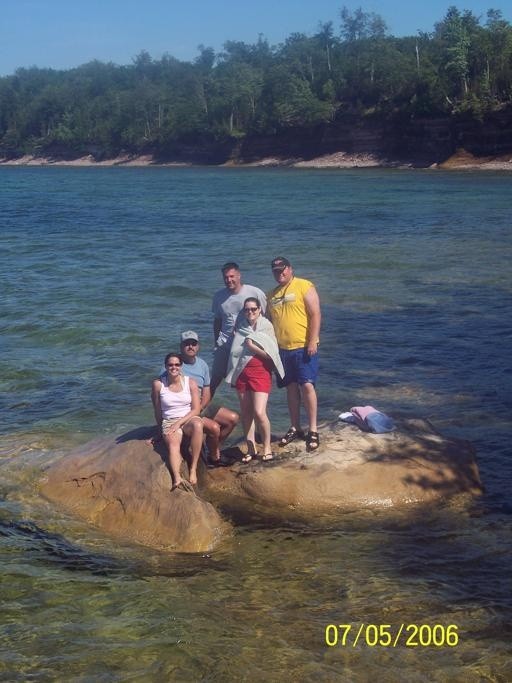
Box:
[207,454,236,468]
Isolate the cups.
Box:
[244,308,257,313]
[167,363,181,367]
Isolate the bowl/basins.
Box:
[271,276,295,299]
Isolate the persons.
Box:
[146,353,204,489]
[209,262,268,401]
[162,331,240,467]
[225,298,285,464]
[265,257,321,451]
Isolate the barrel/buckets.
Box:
[306,431,319,450]
[263,451,275,462]
[282,428,304,446]
[242,451,259,463]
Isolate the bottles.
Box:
[271,256,290,273]
[181,330,199,344]
